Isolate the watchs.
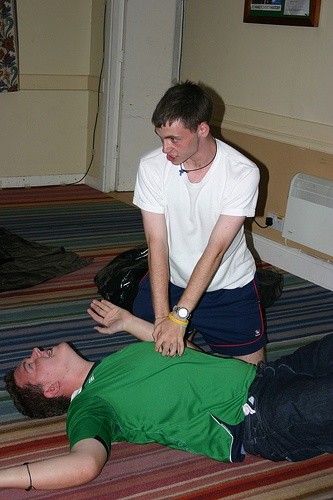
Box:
[173,306,191,320]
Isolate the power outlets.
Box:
[267,214,283,232]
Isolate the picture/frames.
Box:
[242,0,319,27]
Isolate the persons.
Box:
[0,301,333,492]
[132,82,269,366]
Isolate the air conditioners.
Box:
[281,172,333,260]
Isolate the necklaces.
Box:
[179,138,217,176]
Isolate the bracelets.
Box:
[23,462,32,492]
[169,312,189,328]
